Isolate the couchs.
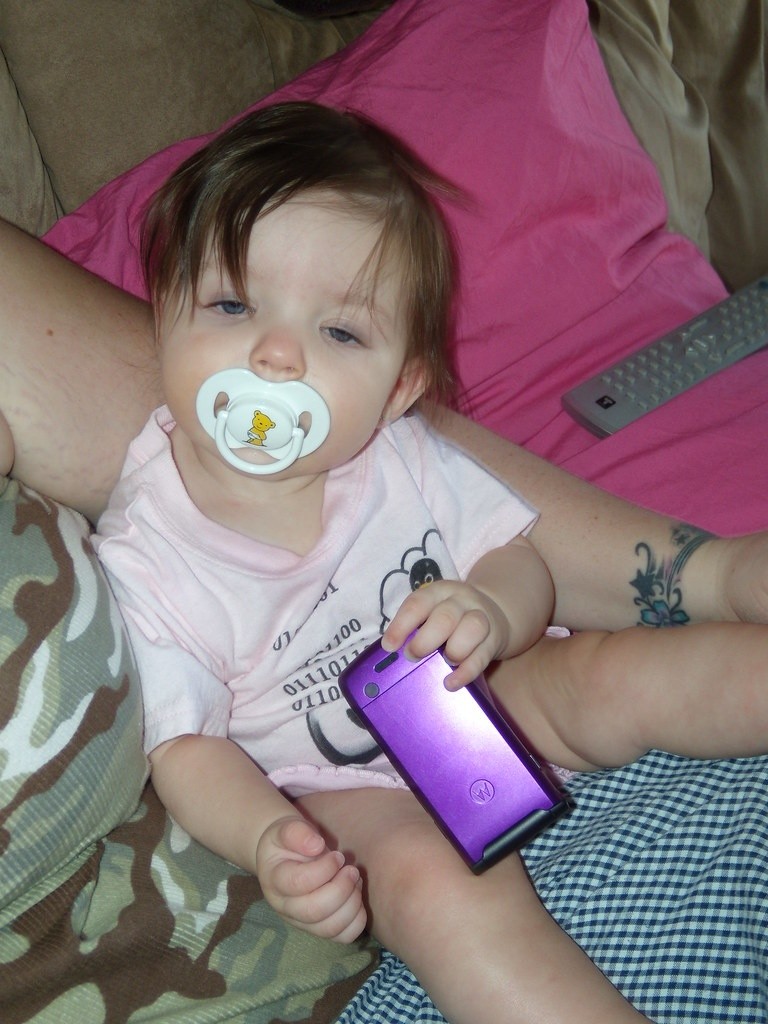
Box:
[0,2,766,547]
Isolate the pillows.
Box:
[38,0,768,538]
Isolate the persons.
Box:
[0,210,768,1024]
[90,102,768,1024]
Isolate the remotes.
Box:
[561,276,768,439]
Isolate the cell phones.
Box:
[335,620,577,876]
